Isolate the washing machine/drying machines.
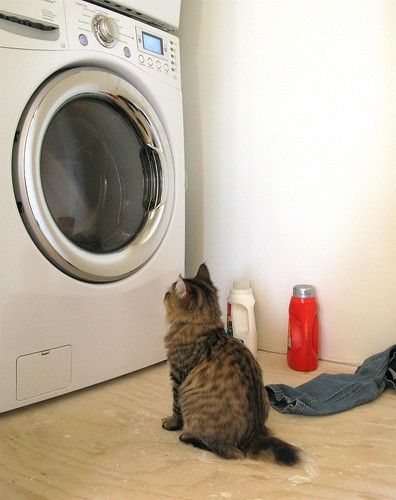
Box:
[0,0,187,414]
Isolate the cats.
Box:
[161,263,308,468]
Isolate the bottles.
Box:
[226,280,258,360]
[286,285,318,372]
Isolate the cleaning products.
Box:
[226,277,260,362]
